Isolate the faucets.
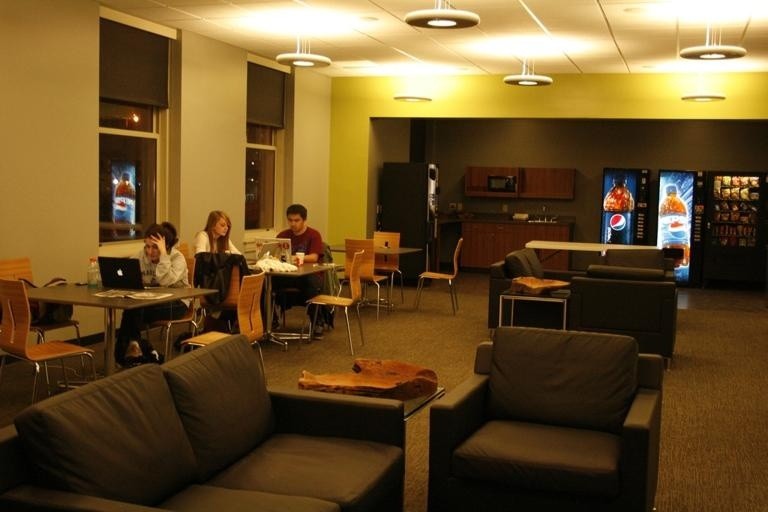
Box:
[542,206,548,222]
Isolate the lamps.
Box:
[273,29,331,69]
[391,94,432,103]
[404,0,480,30]
[677,19,746,60]
[680,92,726,101]
[499,59,552,86]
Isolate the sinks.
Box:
[528,220,557,223]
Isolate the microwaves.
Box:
[487,175,516,192]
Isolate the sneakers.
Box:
[124,338,143,361]
[271,320,281,331]
[312,324,324,340]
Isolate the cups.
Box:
[296,253,304,265]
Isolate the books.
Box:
[91,288,173,302]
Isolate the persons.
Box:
[273,204,327,341]
[189,209,284,333]
[113,221,194,369]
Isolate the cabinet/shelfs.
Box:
[463,166,577,202]
[458,213,574,275]
[700,169,766,291]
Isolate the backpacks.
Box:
[17,277,74,325]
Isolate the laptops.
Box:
[97,255,158,291]
[256,238,295,265]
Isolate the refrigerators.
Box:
[378,160,439,288]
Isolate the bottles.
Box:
[114,173,135,237]
[604,176,634,244]
[660,184,690,270]
[86,257,100,290]
